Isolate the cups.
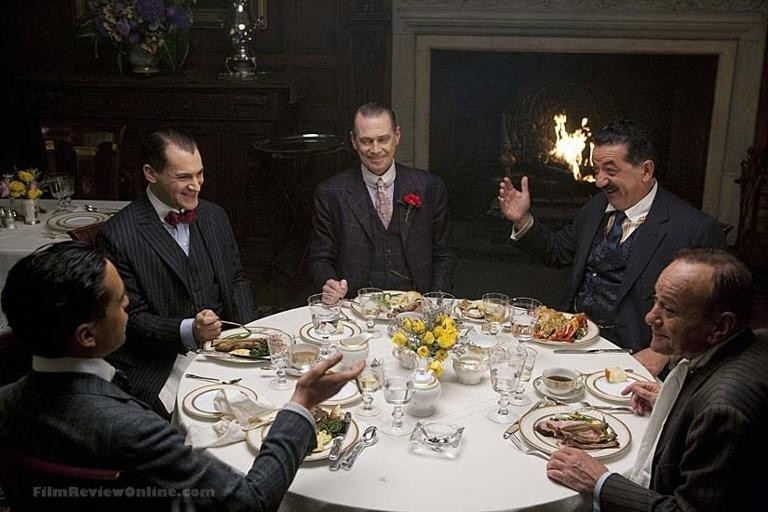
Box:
[542,367,582,394]
[267,287,544,436]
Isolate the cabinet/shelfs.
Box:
[52,80,296,250]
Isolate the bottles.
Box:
[0,207,15,230]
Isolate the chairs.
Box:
[720,140,768,281]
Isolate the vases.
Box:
[21,196,41,225]
[127,47,163,75]
[414,356,440,376]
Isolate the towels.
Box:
[181,387,284,452]
[626,357,691,489]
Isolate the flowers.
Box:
[399,192,424,223]
[382,290,468,377]
[0,169,47,200]
[76,1,197,53]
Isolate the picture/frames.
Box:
[187,1,268,30]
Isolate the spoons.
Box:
[341,425,379,469]
[84,205,120,214]
[572,401,635,412]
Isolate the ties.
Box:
[605,212,627,251]
[375,179,394,229]
[112,369,134,395]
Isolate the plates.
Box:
[531,376,587,400]
[532,312,599,344]
[246,406,360,460]
[46,211,109,231]
[203,326,295,363]
[585,372,652,402]
[520,407,631,460]
[183,384,259,420]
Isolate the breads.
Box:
[605,367,629,383]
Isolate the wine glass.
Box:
[49,176,79,212]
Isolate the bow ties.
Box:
[163,210,198,227]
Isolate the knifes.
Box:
[552,347,633,353]
[502,401,543,438]
[329,409,351,461]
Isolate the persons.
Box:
[311,102,457,308]
[1,241,367,511]
[546,247,768,511]
[498,127,728,378]
[95,128,256,420]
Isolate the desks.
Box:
[252,130,353,254]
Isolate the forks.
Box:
[507,432,552,461]
[217,319,266,333]
[184,372,242,384]
